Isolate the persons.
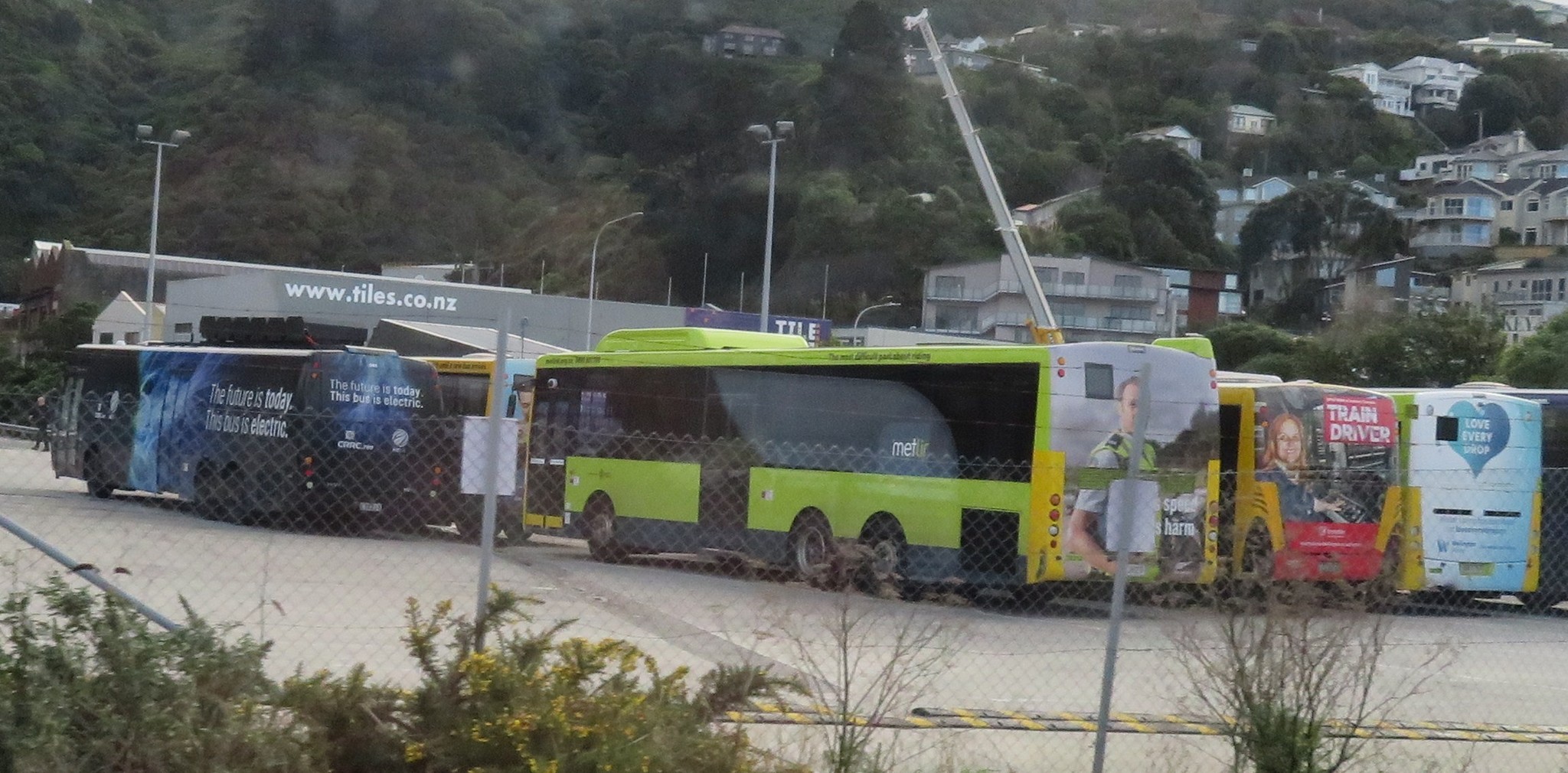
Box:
[1256,414,1345,522]
[29,396,49,451]
[517,386,534,443]
[1067,376,1162,580]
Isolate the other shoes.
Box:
[32,445,49,452]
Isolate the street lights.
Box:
[587,209,645,349]
[852,301,903,347]
[132,123,191,343]
[743,119,795,331]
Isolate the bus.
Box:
[1459,379,1567,611]
[1344,383,1547,607]
[401,351,543,544]
[1207,369,1401,604]
[45,316,456,533]
[517,325,1223,601]
